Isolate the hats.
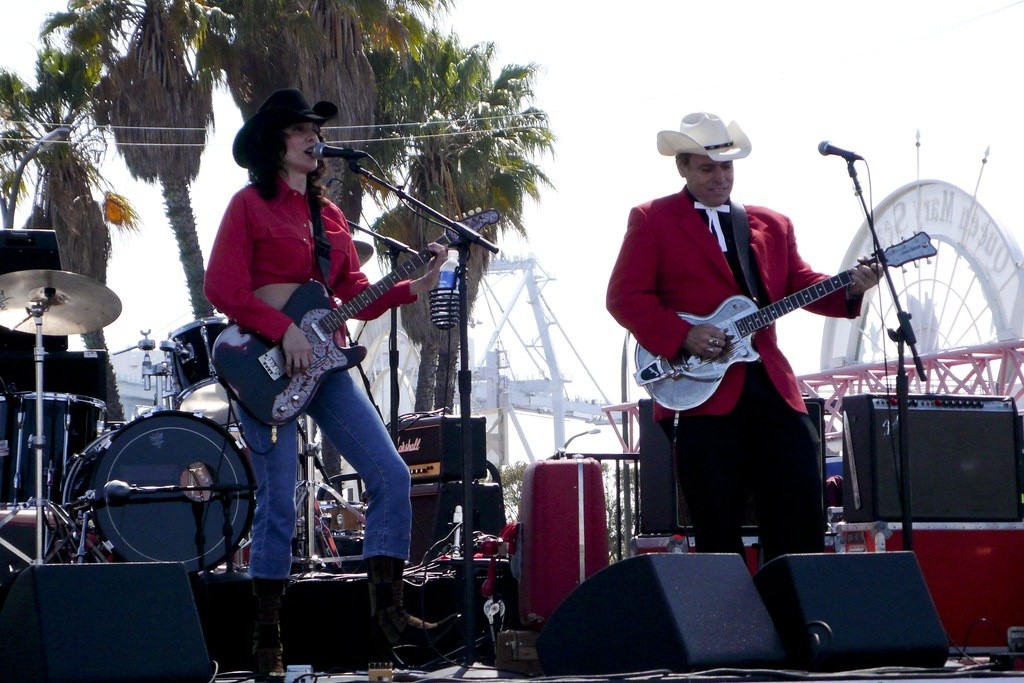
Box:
[233,88,339,168]
[657,112,752,162]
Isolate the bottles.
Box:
[437,249,458,324]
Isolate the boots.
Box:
[367,556,463,667]
[252,577,286,679]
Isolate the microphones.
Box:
[453,505,463,550]
[311,143,367,159]
[817,140,864,161]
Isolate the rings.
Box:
[708,339,719,346]
[706,347,715,353]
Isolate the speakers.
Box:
[638,393,827,534]
[753,551,949,673]
[0,562,214,683]
[535,553,785,676]
[410,482,502,565]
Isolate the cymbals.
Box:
[341,240,374,269]
[0,269,123,335]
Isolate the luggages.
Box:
[518,453,610,631]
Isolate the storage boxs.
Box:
[835,521,1024,654]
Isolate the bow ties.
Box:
[694,201,731,254]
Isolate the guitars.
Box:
[632,230,939,412]
[211,206,500,427]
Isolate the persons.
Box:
[603,112,886,558]
[203,87,462,683]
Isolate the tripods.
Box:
[0,303,109,564]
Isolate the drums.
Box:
[167,315,242,425]
[330,502,368,541]
[62,404,261,573]
[11,391,108,505]
